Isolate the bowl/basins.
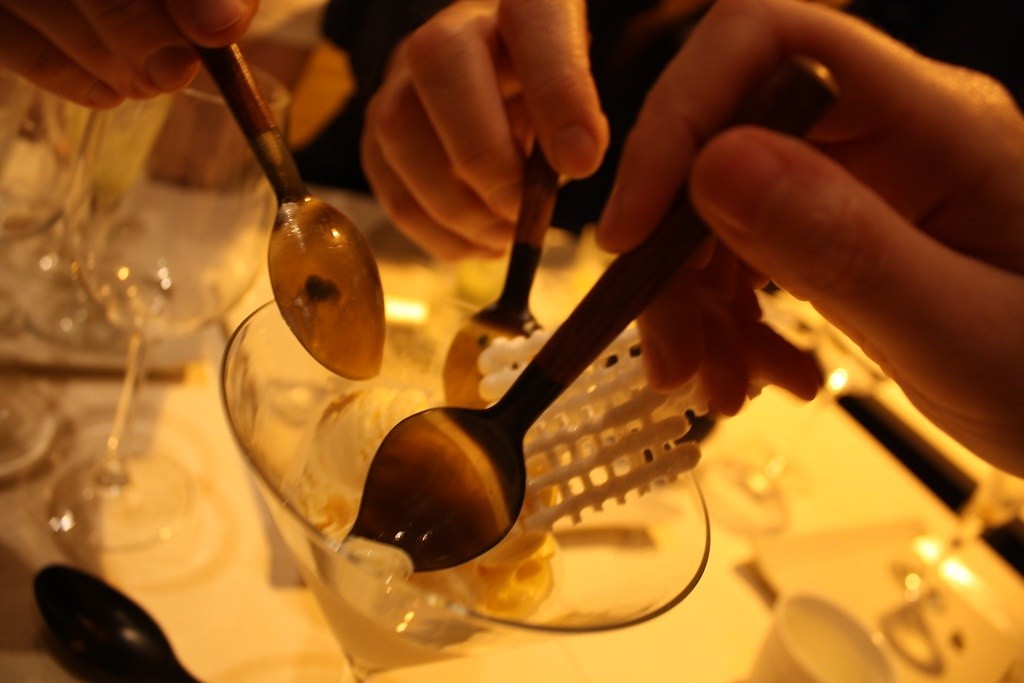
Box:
[218,284,713,648]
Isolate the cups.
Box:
[743,594,898,683]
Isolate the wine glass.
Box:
[886,459,1023,647]
[0,69,274,554]
[712,320,892,529]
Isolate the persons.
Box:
[0,0,1024,477]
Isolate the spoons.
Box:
[31,562,202,682]
[356,50,834,572]
[443,132,559,406]
[196,41,386,380]
